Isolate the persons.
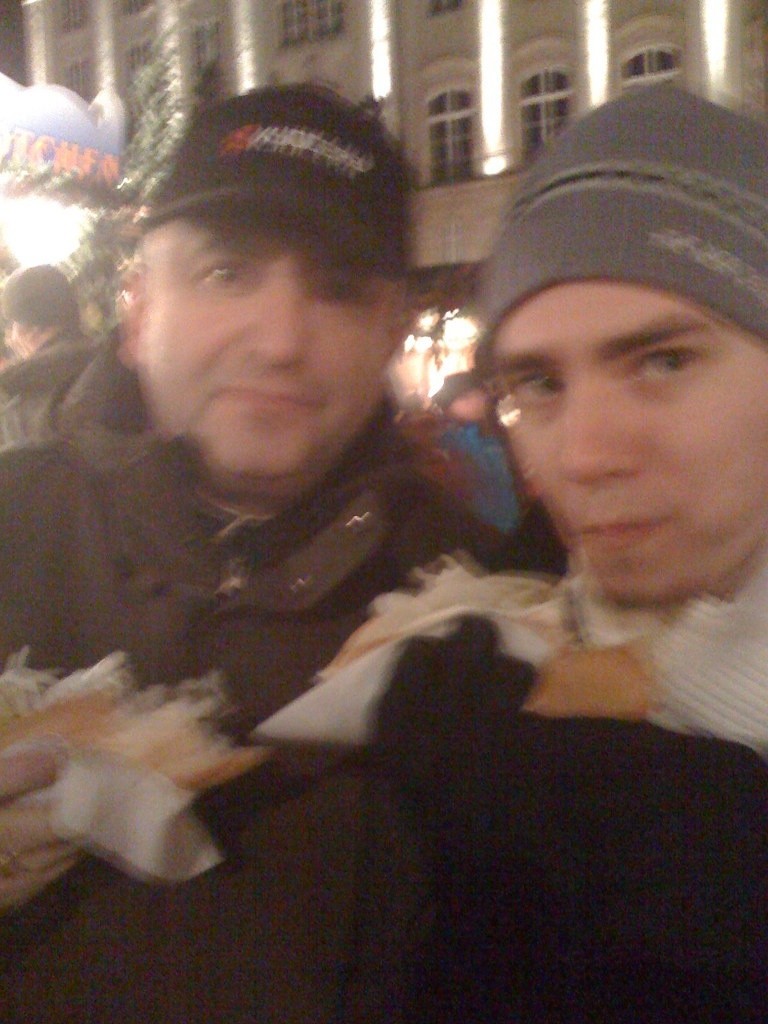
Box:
[440,375,522,533]
[0,264,98,449]
[1,80,497,741]
[0,84,768,1024]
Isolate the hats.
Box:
[132,84,412,278]
[476,83,768,387]
[1,264,80,328]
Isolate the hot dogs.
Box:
[310,557,654,724]
[0,661,275,797]
